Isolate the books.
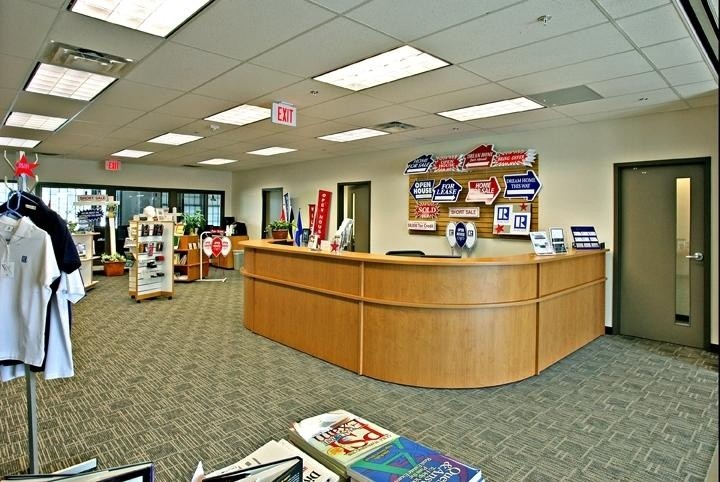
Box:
[0,458,154,481]
[192,408,484,481]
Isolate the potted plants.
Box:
[182,209,206,235]
[264,219,297,239]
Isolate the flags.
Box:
[280,208,303,247]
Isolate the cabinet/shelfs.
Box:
[71,232,101,291]
[128,220,249,303]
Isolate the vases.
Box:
[104,262,124,277]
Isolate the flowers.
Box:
[101,252,127,263]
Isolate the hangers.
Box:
[1,190,61,240]
